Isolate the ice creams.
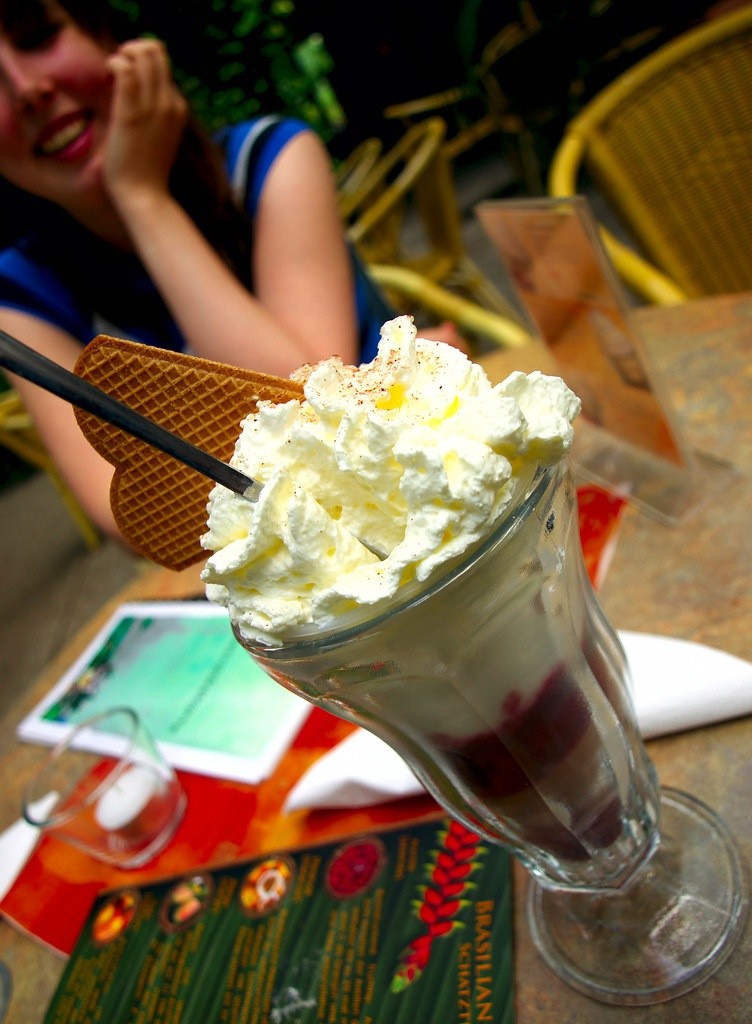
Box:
[65,314,642,860]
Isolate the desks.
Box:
[0,280,749,1022]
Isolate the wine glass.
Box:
[230,433,745,1007]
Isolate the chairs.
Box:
[1,8,748,561]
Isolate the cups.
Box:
[21,707,189,870]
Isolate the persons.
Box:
[0,0,473,555]
